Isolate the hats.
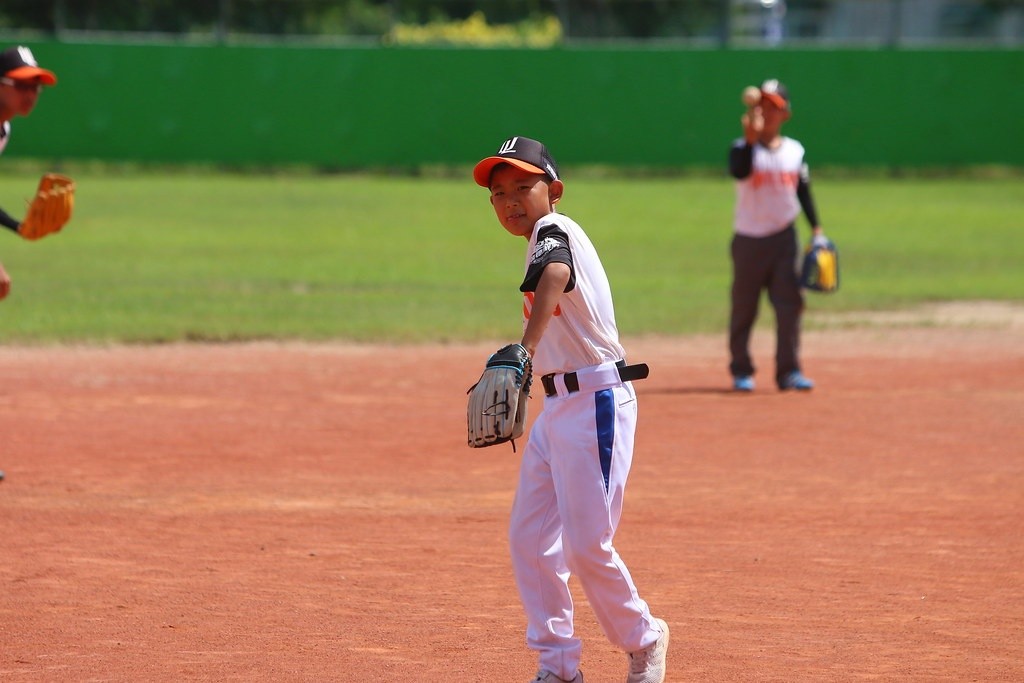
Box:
[759,79,792,111]
[473,135,560,187]
[0,46,57,86]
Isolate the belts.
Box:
[541,358,649,398]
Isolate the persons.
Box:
[468,136,669,683]
[0,45,74,303]
[727,78,838,391]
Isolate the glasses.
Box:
[0,76,39,91]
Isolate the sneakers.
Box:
[779,370,812,390]
[529,670,583,683]
[628,618,669,683]
[733,376,753,391]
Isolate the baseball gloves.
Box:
[21,175,77,240]
[467,343,533,449]
[801,241,840,293]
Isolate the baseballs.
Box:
[743,85,762,106]
